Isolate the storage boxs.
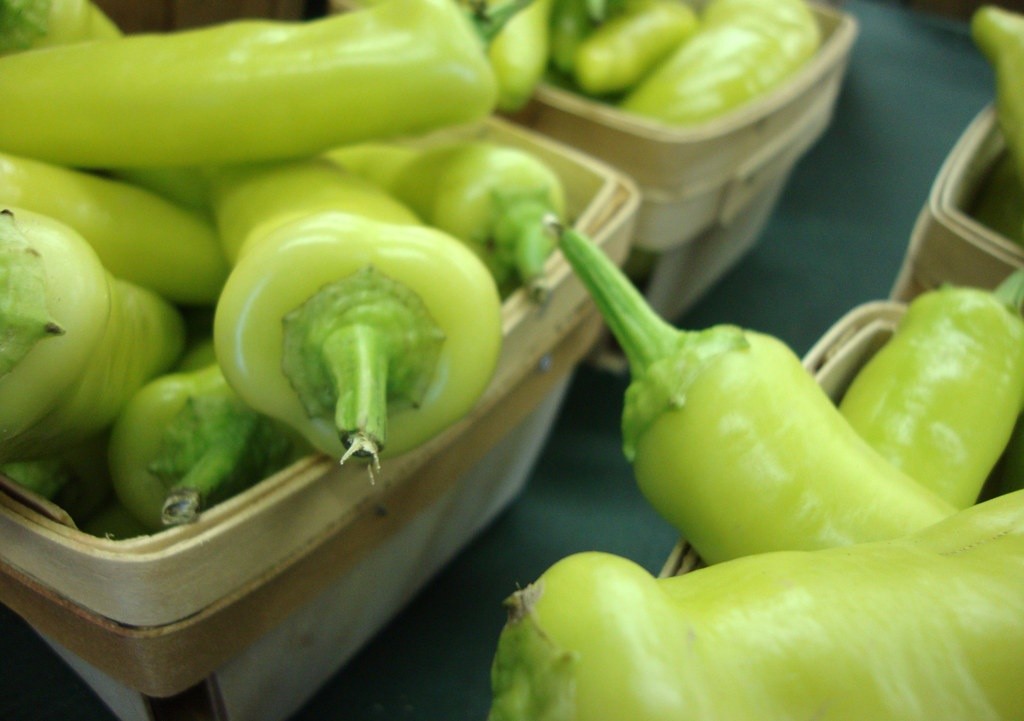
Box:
[889,103,1024,303]
[0,114,644,721]
[652,297,1024,580]
[494,0,859,372]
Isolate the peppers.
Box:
[0,0,829,542]
[483,0,1024,721]
